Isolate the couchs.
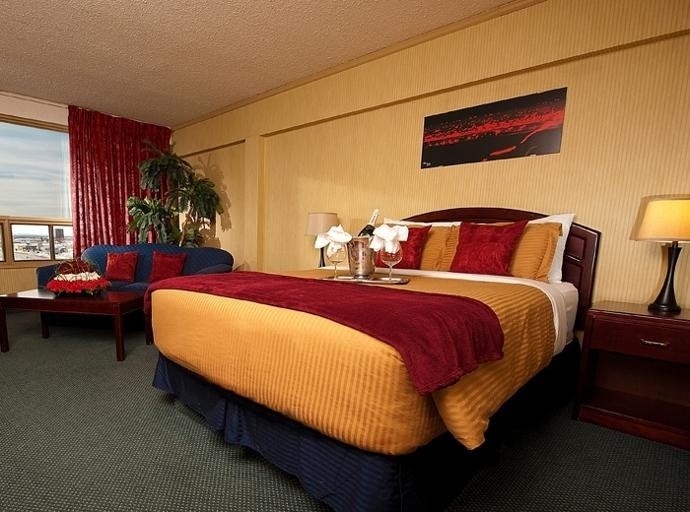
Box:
[35,244,234,331]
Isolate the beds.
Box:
[146,207,602,488]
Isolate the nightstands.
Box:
[572,299,690,449]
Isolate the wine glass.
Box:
[380,245,403,280]
[326,242,347,280]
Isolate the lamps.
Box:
[305,211,337,268]
[636,200,690,315]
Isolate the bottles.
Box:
[358,208,380,236]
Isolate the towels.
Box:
[313,224,354,259]
[367,223,409,254]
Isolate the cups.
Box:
[348,237,375,279]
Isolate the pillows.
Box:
[453,211,576,283]
[439,222,560,284]
[450,218,529,275]
[105,251,139,282]
[380,216,455,271]
[362,225,450,270]
[372,223,432,269]
[150,252,188,281]
